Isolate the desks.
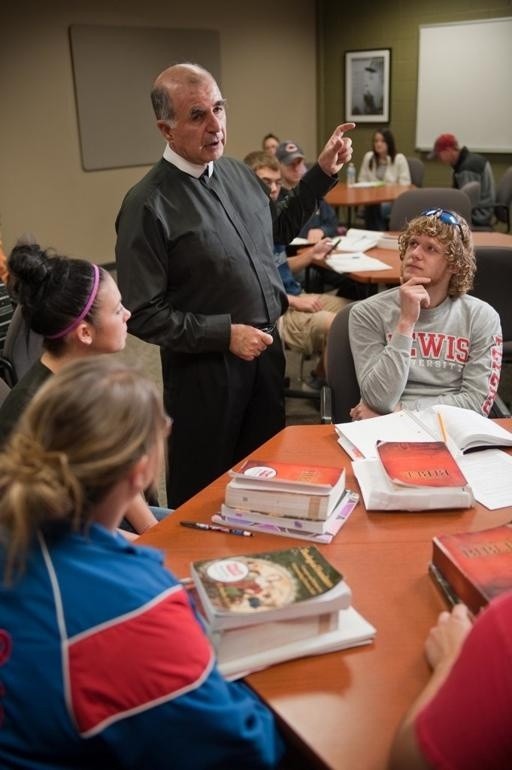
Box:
[129,417,512,769]
[323,183,417,232]
[298,231,512,294]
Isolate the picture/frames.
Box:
[344,47,391,125]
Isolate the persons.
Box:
[349,209,502,418]
[428,134,495,225]
[359,128,411,231]
[245,151,354,395]
[1,233,178,544]
[113,63,355,507]
[388,589,512,769]
[1,351,284,769]
[263,133,346,281]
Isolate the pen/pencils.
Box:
[179,519,254,538]
[429,562,458,609]
[437,411,448,442]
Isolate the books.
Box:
[352,440,475,511]
[428,521,512,619]
[182,545,376,680]
[213,460,359,543]
[335,405,512,460]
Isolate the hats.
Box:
[427,134,458,159]
[275,142,306,166]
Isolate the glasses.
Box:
[418,209,464,243]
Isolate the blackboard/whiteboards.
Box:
[413,15,511,155]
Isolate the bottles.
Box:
[347,161,356,186]
